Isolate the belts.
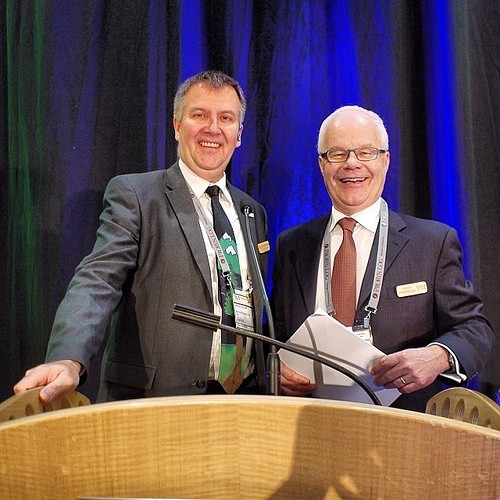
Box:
[207,381,223,392]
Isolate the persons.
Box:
[262,105,497,413]
[12,68,270,405]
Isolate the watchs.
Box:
[442,351,456,375]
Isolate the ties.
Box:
[206,185,250,394]
[329,218,357,327]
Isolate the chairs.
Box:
[0,386,91,426]
[425,386,498,432]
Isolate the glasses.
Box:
[321,146,385,162]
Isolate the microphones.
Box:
[171,303,381,406]
[239,197,280,394]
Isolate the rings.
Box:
[399,376,407,385]
